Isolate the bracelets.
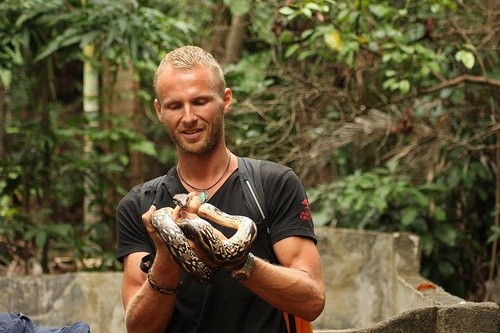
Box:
[147,269,183,296]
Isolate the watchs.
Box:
[229,252,254,281]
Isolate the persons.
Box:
[116,46,325,333]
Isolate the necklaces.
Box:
[176,152,232,204]
